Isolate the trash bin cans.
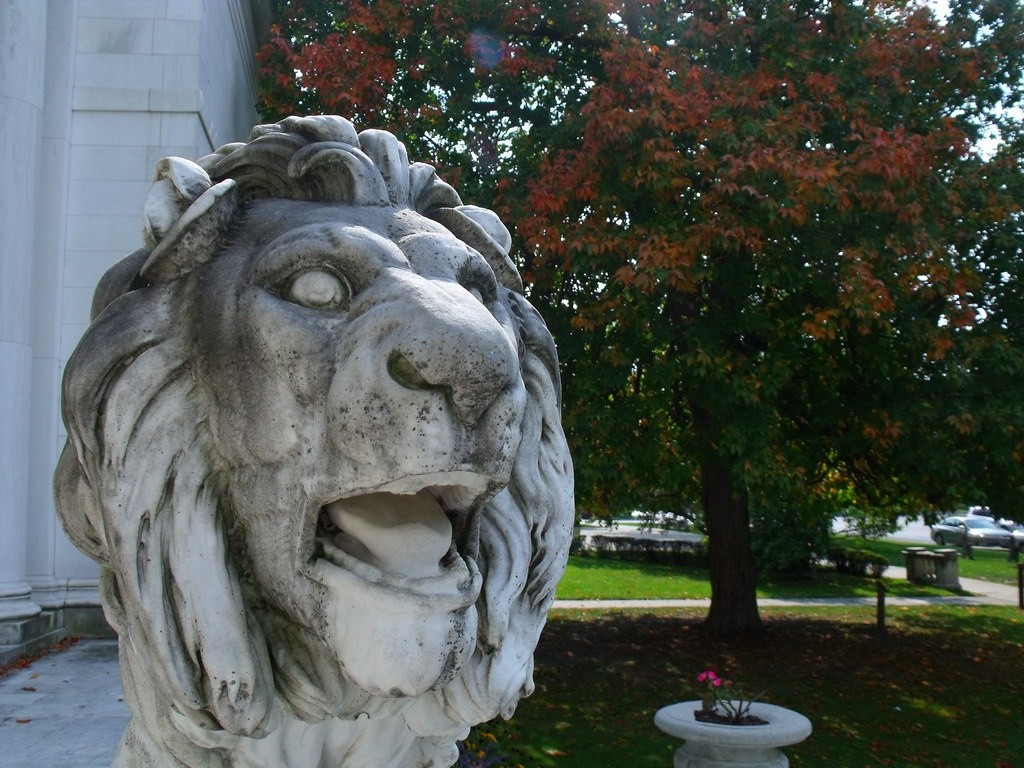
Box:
[931,548,962,590]
[904,546,928,584]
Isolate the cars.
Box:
[931,515,1015,550]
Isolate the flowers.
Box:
[696,671,759,723]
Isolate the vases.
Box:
[653,701,812,768]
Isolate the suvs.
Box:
[966,506,1024,551]
